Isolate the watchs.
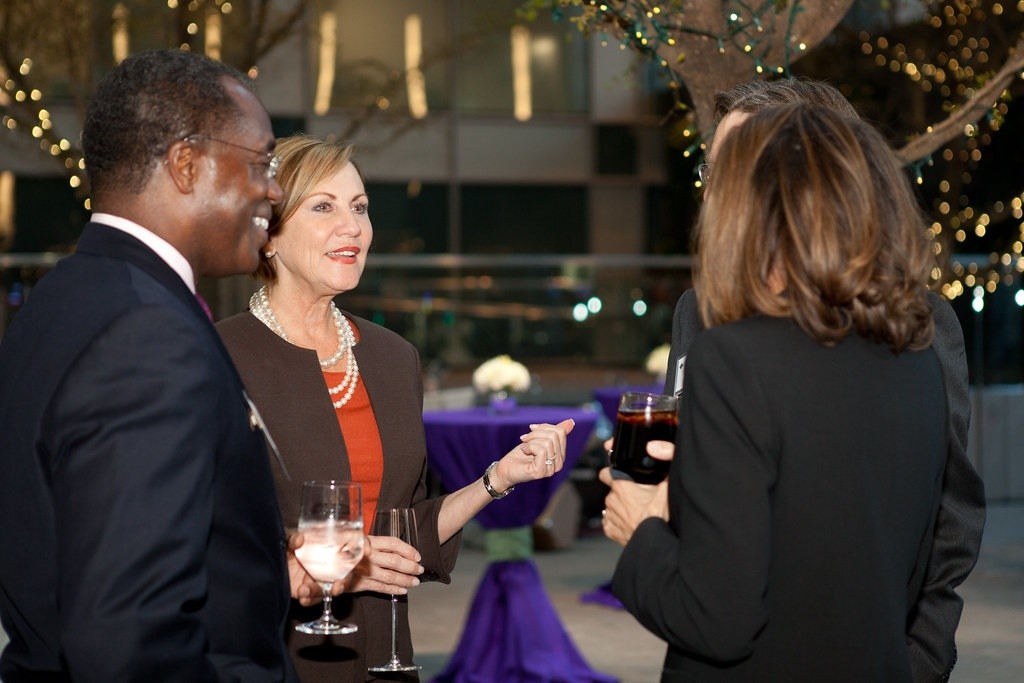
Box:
[483,461,515,499]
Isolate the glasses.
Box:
[154,133,281,179]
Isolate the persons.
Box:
[215,136,574,683]
[598,80,986,683]
[0,52,371,683]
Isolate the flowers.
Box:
[645,343,671,382]
[475,355,532,397]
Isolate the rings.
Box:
[545,453,556,465]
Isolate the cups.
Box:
[607,391,680,482]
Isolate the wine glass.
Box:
[294,480,365,635]
[367,509,425,672]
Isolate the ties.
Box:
[194,293,216,325]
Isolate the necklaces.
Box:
[249,285,359,410]
